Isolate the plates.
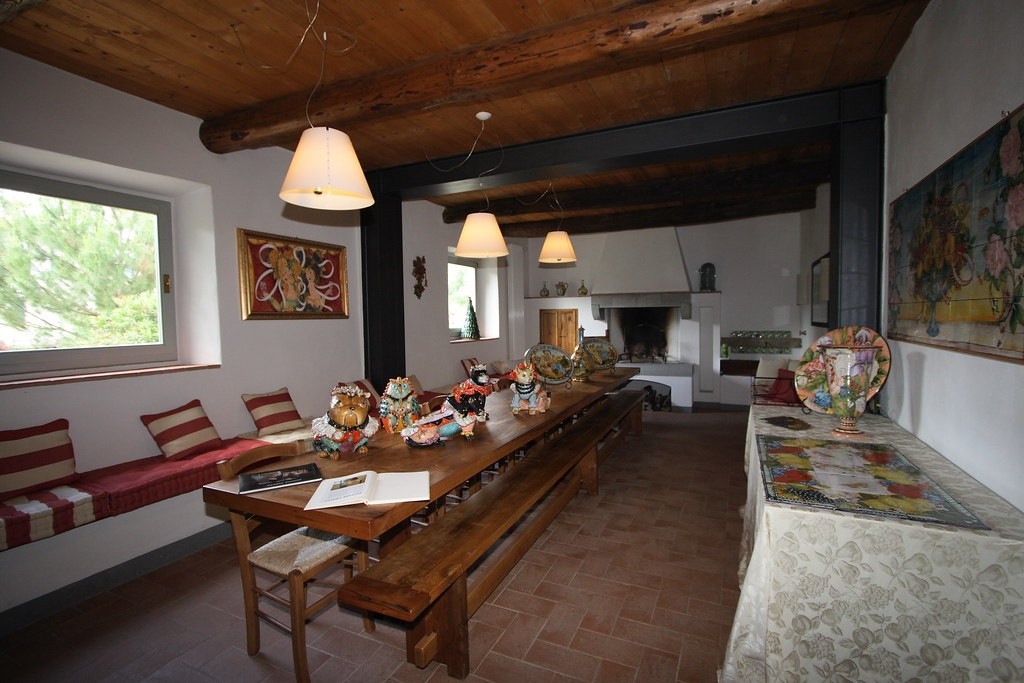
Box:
[576,340,619,368]
[795,326,891,413]
[524,345,574,385]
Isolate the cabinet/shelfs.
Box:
[539,308,578,358]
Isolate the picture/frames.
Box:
[809,251,830,328]
[235,227,350,320]
[886,102,1024,366]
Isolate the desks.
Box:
[717,405,1024,683]
[202,368,640,662]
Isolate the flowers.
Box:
[412,256,428,300]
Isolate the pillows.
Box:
[461,356,480,379]
[139,398,226,463]
[786,359,800,371]
[756,358,788,379]
[339,379,383,414]
[491,361,512,376]
[765,369,799,403]
[242,385,306,439]
[0,417,80,505]
[408,375,424,395]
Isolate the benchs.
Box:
[333,390,649,679]
[751,368,802,405]
[0,380,467,553]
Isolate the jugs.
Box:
[556,282,568,296]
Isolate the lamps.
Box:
[228,0,375,213]
[423,110,510,260]
[515,182,576,264]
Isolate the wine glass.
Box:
[818,344,883,435]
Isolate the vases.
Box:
[539,280,550,297]
[819,342,883,439]
[577,280,588,296]
[555,282,568,296]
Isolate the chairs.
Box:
[494,379,559,454]
[417,394,496,505]
[212,440,376,683]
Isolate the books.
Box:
[238,462,323,495]
[303,470,431,511]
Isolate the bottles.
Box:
[568,324,596,381]
[540,282,549,297]
[578,279,588,295]
